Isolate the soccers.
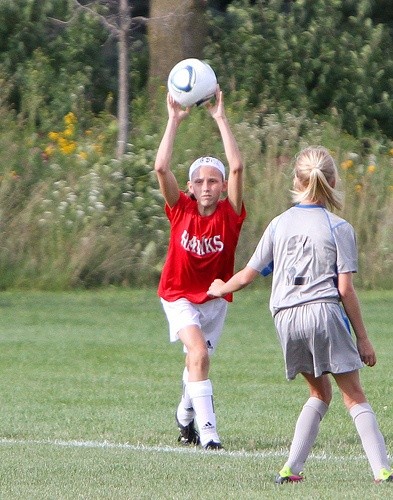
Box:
[167,58,217,108]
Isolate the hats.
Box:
[188,156,226,182]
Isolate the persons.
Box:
[155,84,246,451]
[207,146,393,482]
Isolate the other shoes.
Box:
[199,420,222,448]
[374,467,393,483]
[274,469,304,485]
[175,409,201,446]
